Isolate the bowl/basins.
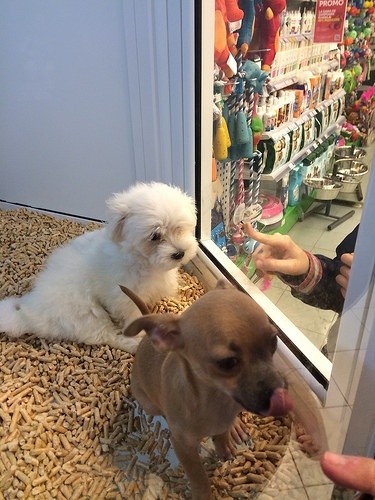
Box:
[332,146,367,161]
[323,176,360,193]
[333,158,369,181]
[303,178,344,200]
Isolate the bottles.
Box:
[252,87,306,131]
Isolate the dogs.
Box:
[116,278,330,500]
[0,178,200,355]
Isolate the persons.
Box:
[245,223,360,362]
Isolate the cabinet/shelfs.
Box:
[213,42,348,280]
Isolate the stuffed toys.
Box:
[338,0,375,146]
[215,0,286,92]
